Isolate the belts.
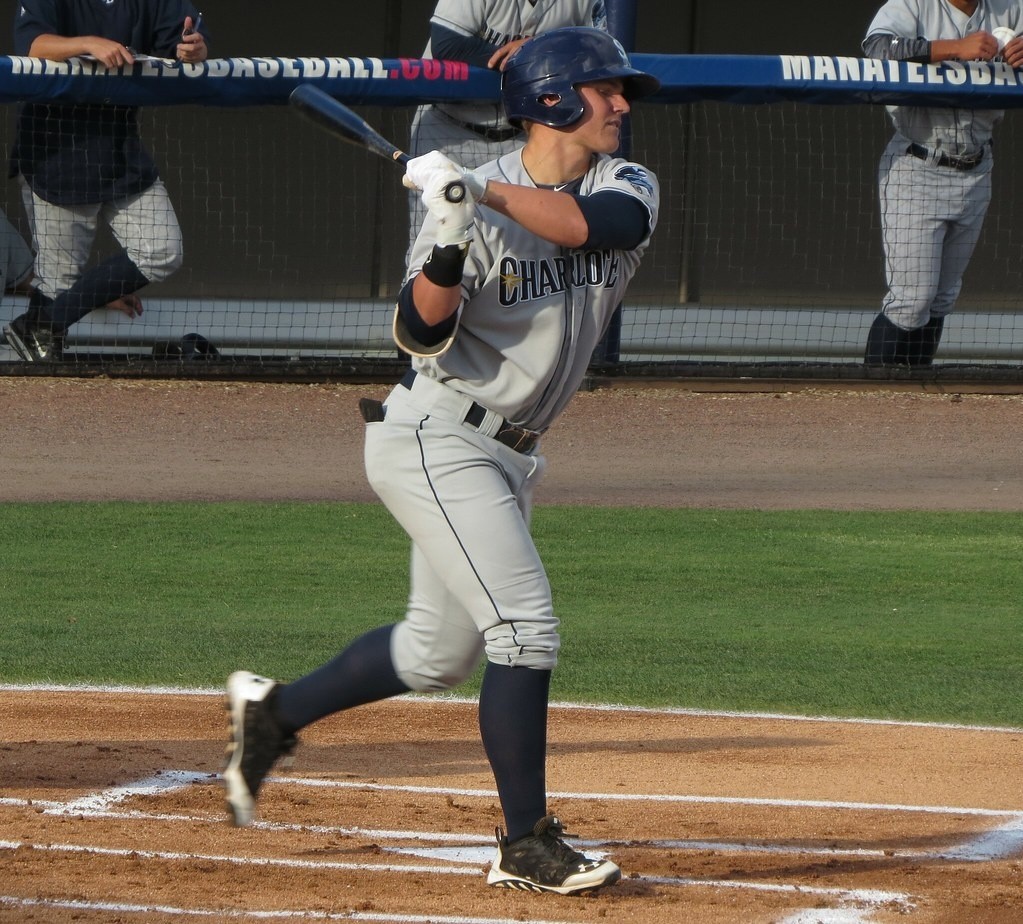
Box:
[433,107,518,143]
[907,142,983,172]
[401,368,538,454]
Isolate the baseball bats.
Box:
[287,79,468,207]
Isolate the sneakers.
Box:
[4,314,66,363]
[486,815,620,896]
[224,669,298,828]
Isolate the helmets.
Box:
[501,27,660,130]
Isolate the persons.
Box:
[2,0,213,362]
[861,0,1023,381]
[405,0,611,268]
[222,25,661,894]
[0,207,144,318]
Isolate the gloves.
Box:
[400,151,487,206]
[420,168,475,250]
